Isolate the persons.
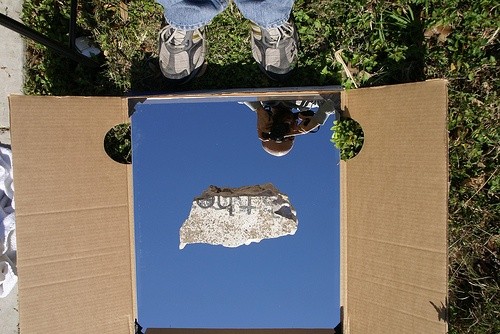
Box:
[153,0,300,84]
[232,101,337,158]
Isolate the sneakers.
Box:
[248,20,299,81]
[158,14,206,86]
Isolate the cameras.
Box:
[261,121,294,143]
